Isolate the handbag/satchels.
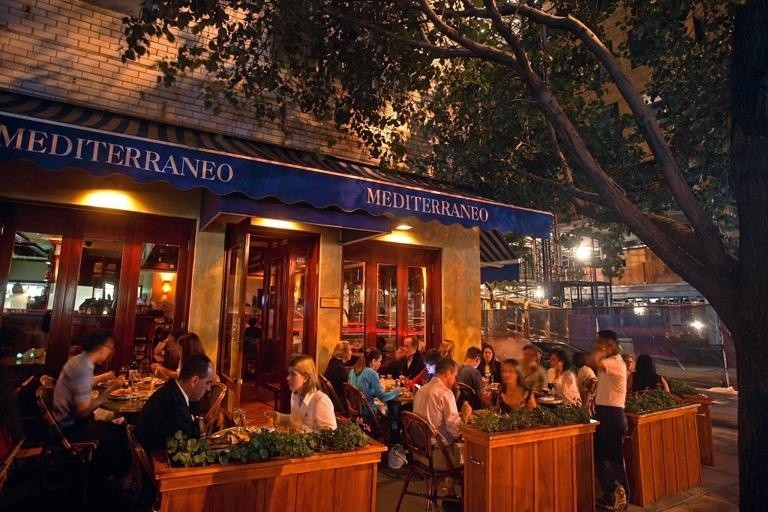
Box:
[388,444,408,468]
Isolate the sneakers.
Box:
[596,485,626,512]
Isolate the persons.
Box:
[244,318,261,339]
[324,331,671,512]
[48,325,215,512]
[9,283,28,309]
[263,355,338,436]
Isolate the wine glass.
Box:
[232,408,246,436]
[377,373,418,398]
[545,376,555,396]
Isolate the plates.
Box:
[94,367,166,402]
[536,392,564,405]
[244,424,276,434]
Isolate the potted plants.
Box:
[625,390,702,508]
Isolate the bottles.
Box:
[128,352,138,380]
[28,347,37,359]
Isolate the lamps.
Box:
[393,221,414,232]
